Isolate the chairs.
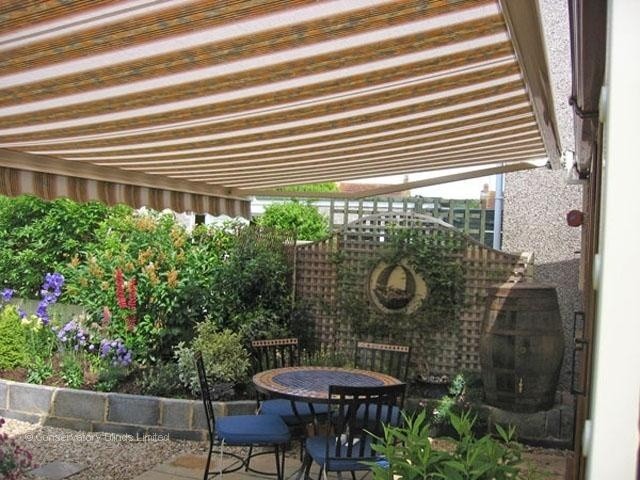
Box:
[195,339,409,480]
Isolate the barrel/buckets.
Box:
[478,284,565,413]
